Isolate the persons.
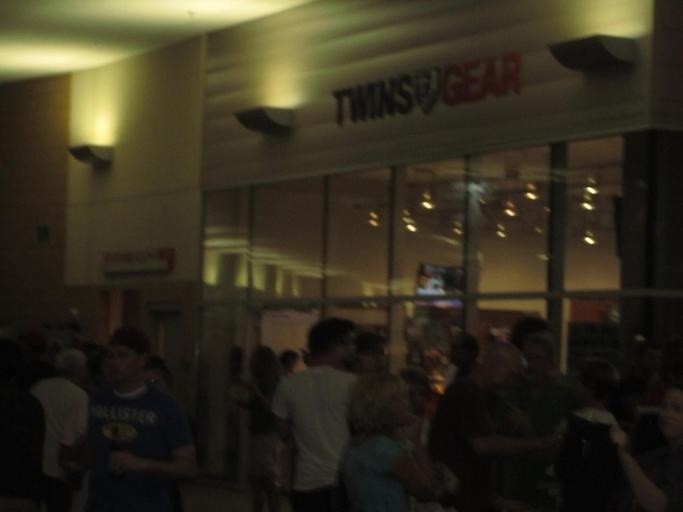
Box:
[1,326,196,512]
[232,315,682,510]
